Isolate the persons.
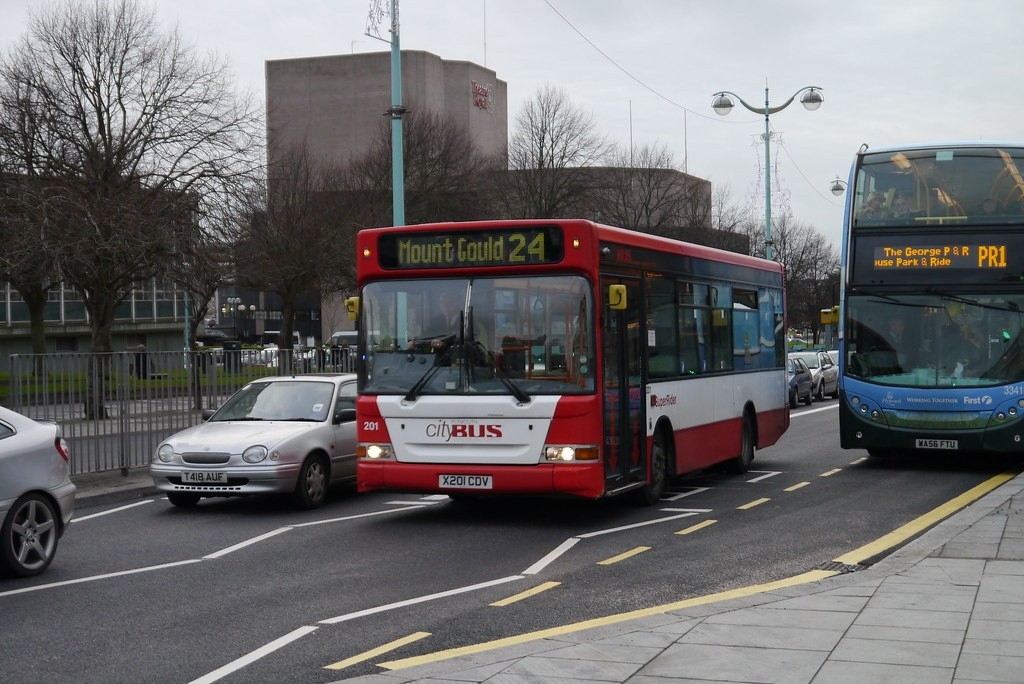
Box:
[135,345,156,380]
[314,338,351,372]
[875,314,909,354]
[854,191,886,221]
[408,288,489,363]
[884,194,922,219]
[981,196,1004,223]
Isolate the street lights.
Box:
[227,297,241,336]
[830,176,875,196]
[711,76,824,261]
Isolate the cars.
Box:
[149,372,358,512]
[788,357,813,409]
[240,330,381,368]
[0,406,77,579]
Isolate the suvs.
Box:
[788,350,838,402]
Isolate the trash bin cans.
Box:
[223,340,243,373]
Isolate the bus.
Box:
[343,218,791,507]
[838,143,1023,469]
